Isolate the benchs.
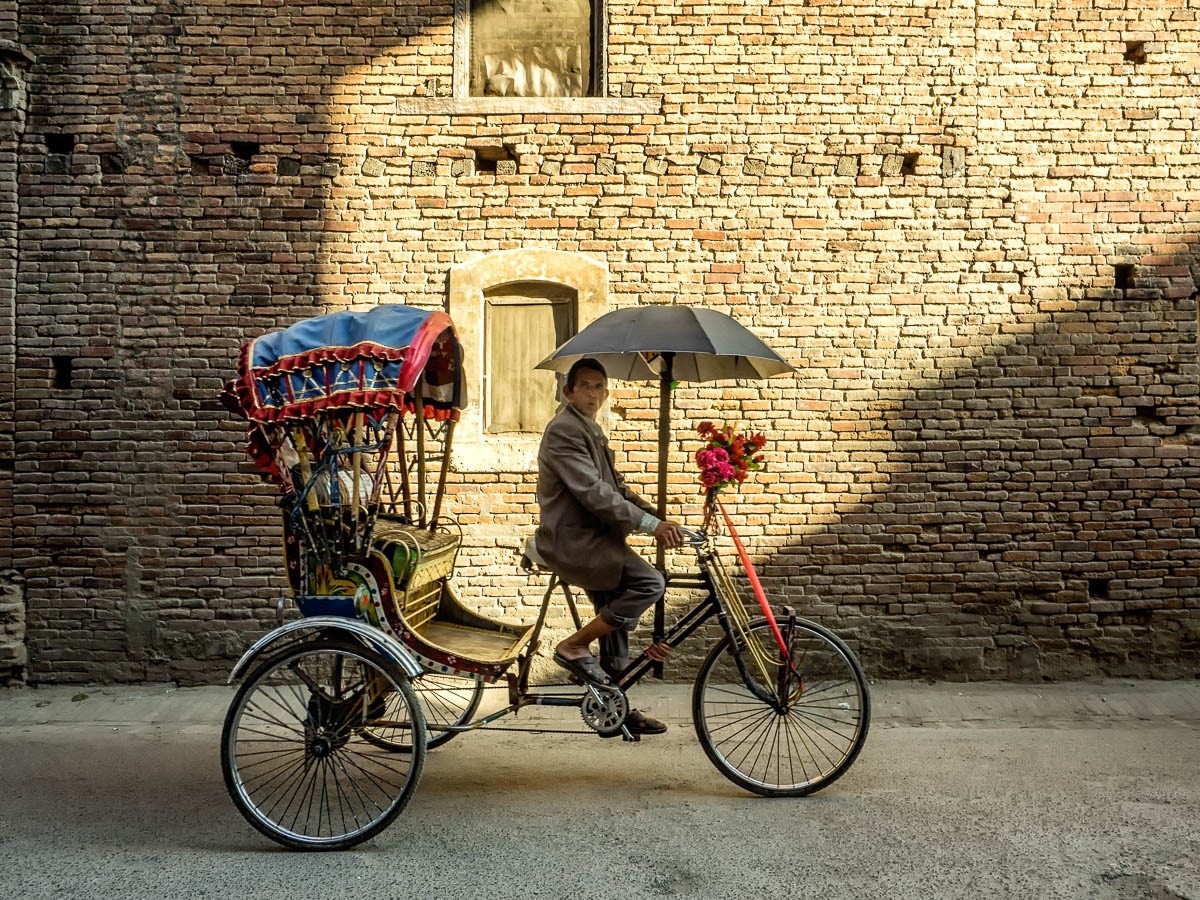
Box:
[317,466,465,591]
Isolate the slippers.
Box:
[553,652,611,685]
[597,708,667,739]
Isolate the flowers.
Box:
[692,419,770,496]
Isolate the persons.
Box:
[533,359,686,739]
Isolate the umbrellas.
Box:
[532,307,800,680]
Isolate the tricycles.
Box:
[217,303,870,851]
